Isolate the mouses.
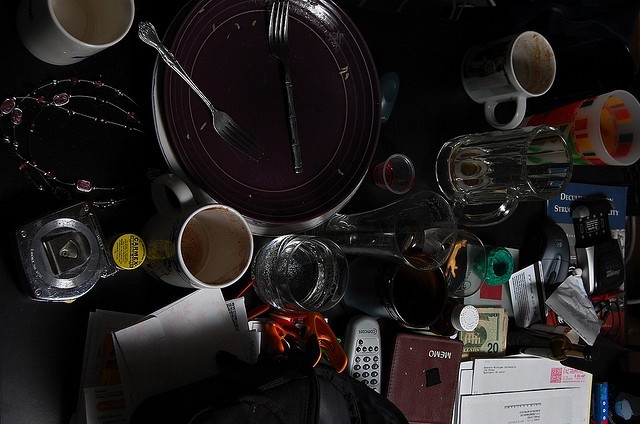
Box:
[517,217,570,305]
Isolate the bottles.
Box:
[400,296,479,340]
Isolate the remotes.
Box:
[338,315,382,396]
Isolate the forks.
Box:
[139,21,266,163]
[268,2,307,174]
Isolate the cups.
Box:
[461,31,556,129]
[512,90,640,165]
[140,173,254,291]
[435,123,573,226]
[17,0,134,66]
[252,234,350,313]
[316,192,457,271]
[413,229,488,300]
[373,153,416,194]
[341,253,447,330]
